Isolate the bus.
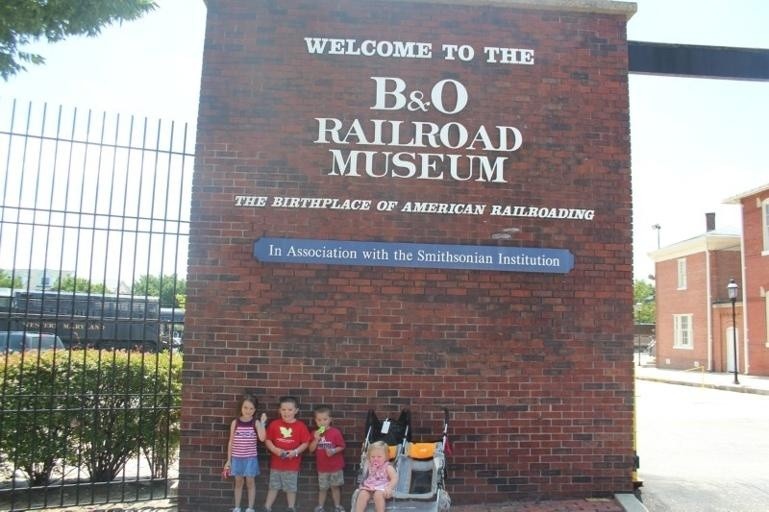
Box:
[1,288,160,354]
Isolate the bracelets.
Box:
[331,448,337,455]
[260,421,265,427]
[293,449,299,458]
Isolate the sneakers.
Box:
[232,507,256,512]
[315,504,345,512]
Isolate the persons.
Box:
[308,405,348,512]
[354,439,399,511]
[223,394,269,512]
[256,395,313,512]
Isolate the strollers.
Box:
[351,408,452,512]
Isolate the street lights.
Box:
[651,223,661,248]
[636,303,642,366]
[727,277,740,385]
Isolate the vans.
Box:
[1,329,64,351]
